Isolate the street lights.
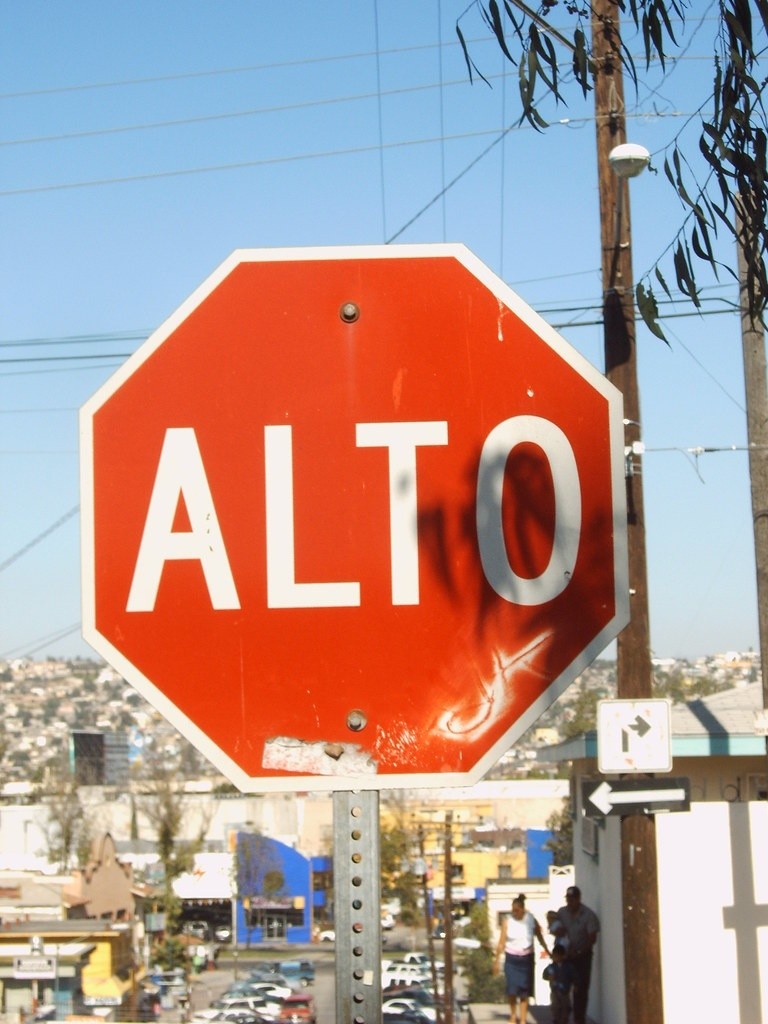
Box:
[590,144,669,1023]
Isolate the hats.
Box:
[565,886,582,898]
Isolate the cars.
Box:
[192,960,317,1024]
[381,955,453,1024]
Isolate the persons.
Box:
[547,886,600,1024]
[491,894,553,1024]
